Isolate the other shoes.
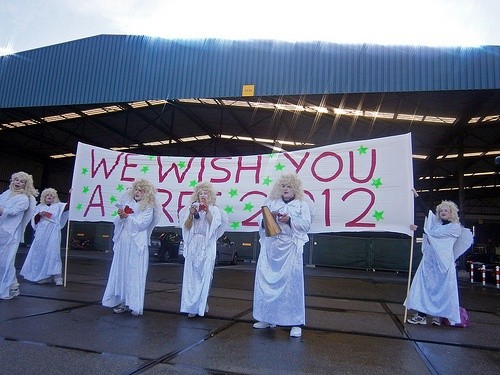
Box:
[37,278,52,284]
[114,305,128,313]
[253,321,276,328]
[54,276,63,286]
[290,327,301,337]
[1,289,21,300]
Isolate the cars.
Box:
[471,262,500,283]
[69,232,95,251]
[466,254,500,273]
[178,233,239,266]
[149,231,184,262]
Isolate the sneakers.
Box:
[431,317,441,326]
[407,314,427,325]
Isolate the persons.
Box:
[101,179,154,314]
[178,181,222,318]
[0,171,40,300]
[402,187,474,326]
[19,187,72,286]
[252,172,311,338]
